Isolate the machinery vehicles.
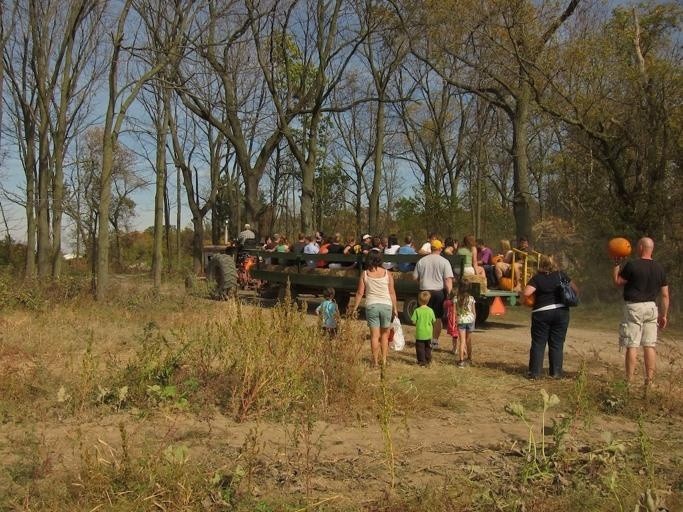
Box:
[181,219,267,299]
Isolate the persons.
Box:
[442,287,466,354]
[263,232,493,277]
[612,237,671,388]
[452,278,477,368]
[238,223,255,247]
[350,249,398,367]
[314,288,341,340]
[410,296,436,367]
[412,240,454,351]
[522,255,579,381]
[514,236,529,260]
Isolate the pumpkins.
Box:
[524,295,534,306]
[609,239,632,260]
[499,277,516,290]
[492,256,503,265]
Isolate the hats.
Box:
[363,234,371,239]
[431,239,445,251]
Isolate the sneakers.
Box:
[417,344,473,369]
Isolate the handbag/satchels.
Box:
[559,282,582,307]
[393,314,405,352]
